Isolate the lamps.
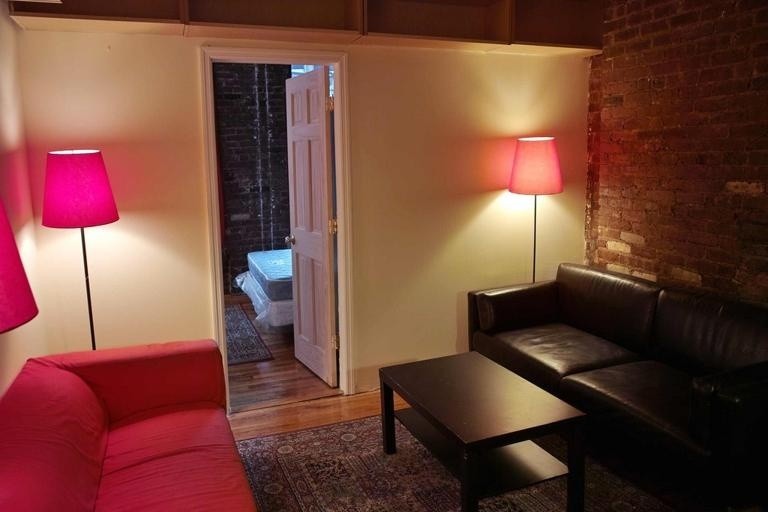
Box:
[0,202,39,336]
[507,131,565,283]
[39,146,120,351]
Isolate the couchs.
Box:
[465,266,767,511]
[3,342,249,511]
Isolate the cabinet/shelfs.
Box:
[184,0,359,47]
[8,0,189,39]
[495,0,605,56]
[351,0,510,53]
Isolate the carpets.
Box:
[230,402,679,510]
[223,301,273,366]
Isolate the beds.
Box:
[237,249,293,339]
